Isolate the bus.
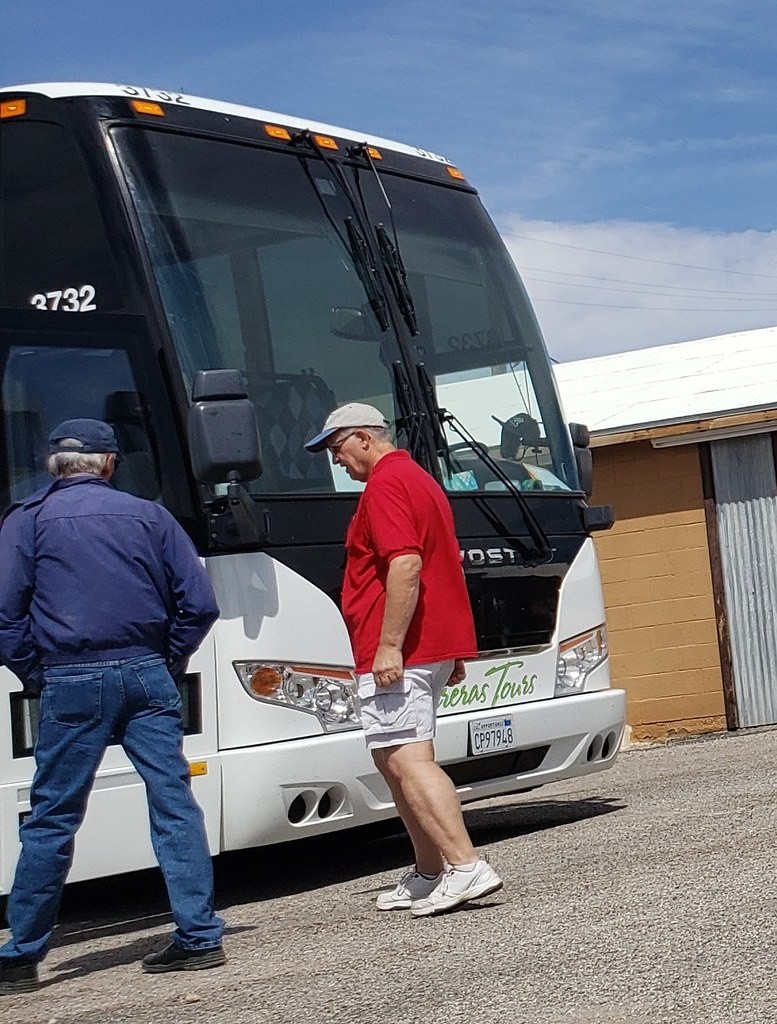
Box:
[0,76,631,899]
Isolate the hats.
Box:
[45,417,128,467]
[302,402,390,456]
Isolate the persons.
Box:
[0,417,230,992]
[302,401,505,918]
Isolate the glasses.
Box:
[327,430,372,455]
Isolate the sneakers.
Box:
[375,867,447,910]
[409,856,507,918]
[140,934,229,972]
[0,949,41,995]
